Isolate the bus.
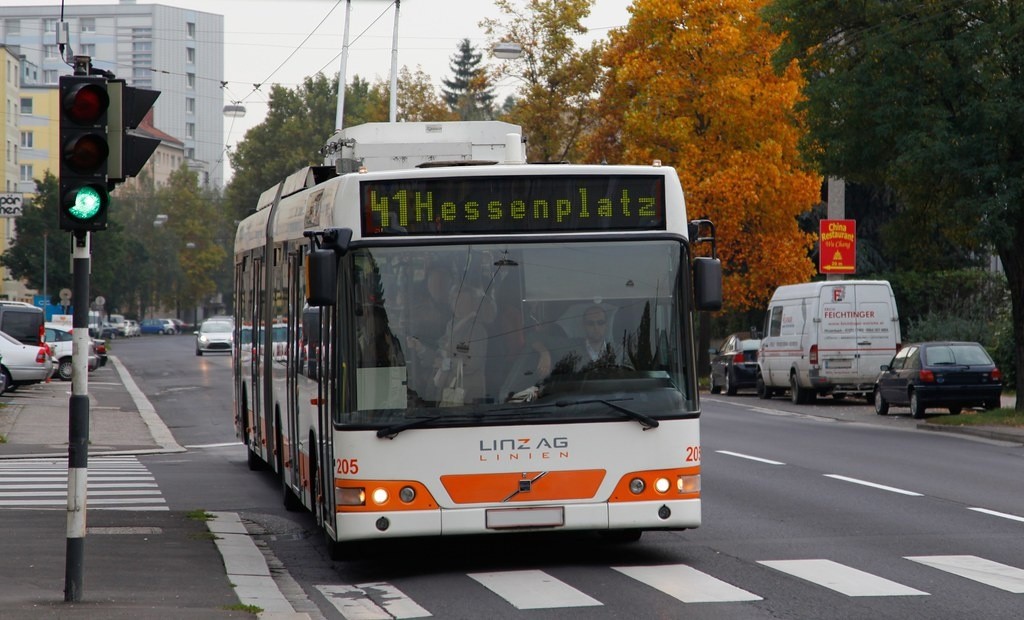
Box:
[230,120,725,564]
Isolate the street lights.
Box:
[174,242,196,319]
[151,214,168,319]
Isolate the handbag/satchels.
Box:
[439,360,464,407]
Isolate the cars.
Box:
[1,299,309,397]
[871,340,1003,419]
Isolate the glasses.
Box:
[584,320,606,326]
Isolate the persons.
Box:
[564,305,634,370]
[358,251,553,407]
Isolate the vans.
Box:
[752,280,904,407]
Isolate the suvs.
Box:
[708,331,764,396]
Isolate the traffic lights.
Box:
[108,78,163,181]
[60,76,110,232]
